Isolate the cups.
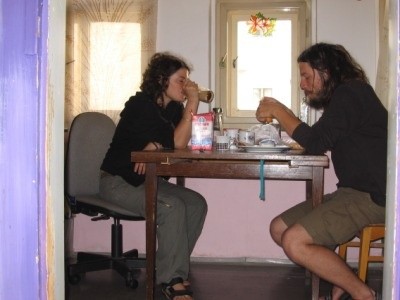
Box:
[215,127,254,151]
[181,85,214,103]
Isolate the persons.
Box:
[99,53,208,300]
[255,41,388,300]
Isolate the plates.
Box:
[238,145,291,152]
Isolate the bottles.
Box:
[213,107,224,146]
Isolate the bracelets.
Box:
[186,107,194,116]
[153,142,158,151]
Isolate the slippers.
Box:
[320,289,350,300]
[375,291,382,300]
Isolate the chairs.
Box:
[337,223,385,284]
[66,111,145,290]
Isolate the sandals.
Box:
[161,276,194,300]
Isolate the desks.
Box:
[131,148,329,300]
